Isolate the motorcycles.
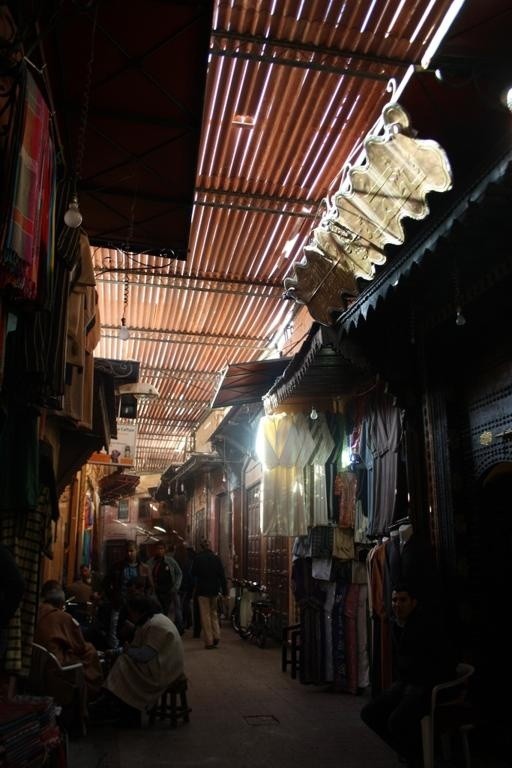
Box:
[232,575,272,647]
[61,595,111,659]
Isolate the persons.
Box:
[146,540,230,650]
[358,584,451,767]
[31,540,184,739]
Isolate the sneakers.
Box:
[204,644,214,649]
[212,638,220,646]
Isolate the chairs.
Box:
[1,603,193,766]
